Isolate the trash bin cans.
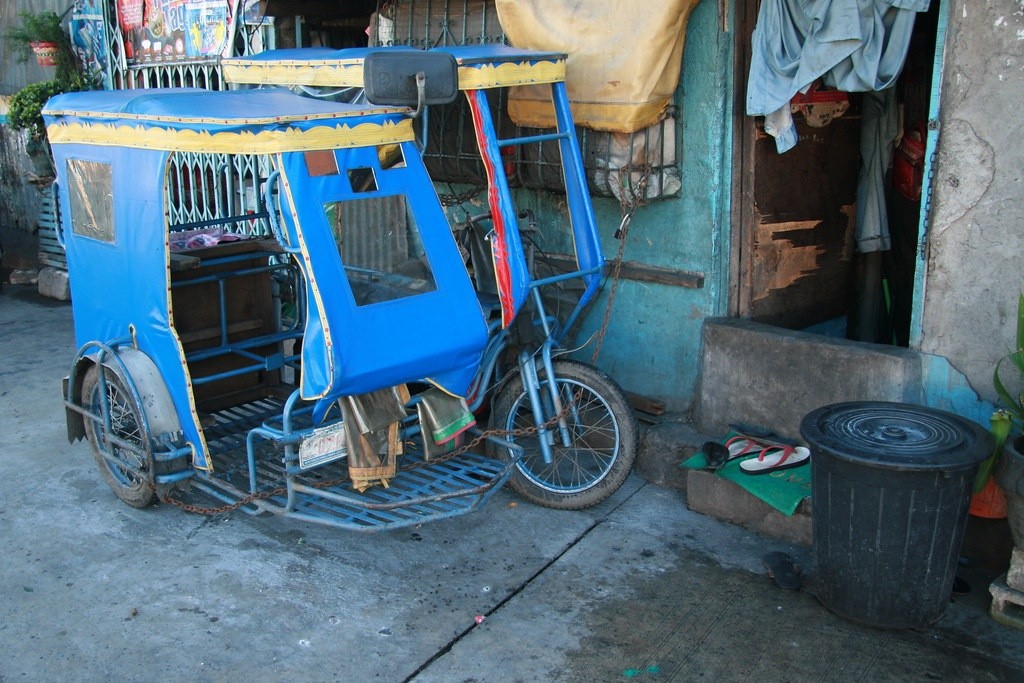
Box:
[798,400,996,631]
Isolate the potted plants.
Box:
[0,10,71,66]
[972,293,1024,553]
[9,80,61,176]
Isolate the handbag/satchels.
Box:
[892,121,926,201]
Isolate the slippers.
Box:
[725,435,766,462]
[740,444,810,475]
[702,441,730,469]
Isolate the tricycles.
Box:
[41,46,639,534]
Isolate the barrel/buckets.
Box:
[801,402,995,629]
